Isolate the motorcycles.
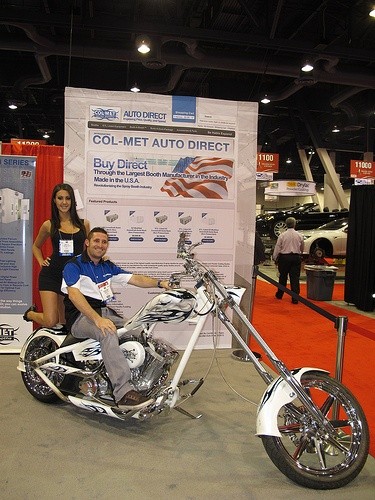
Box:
[16,235,370,491]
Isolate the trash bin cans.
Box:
[304,265,338,301]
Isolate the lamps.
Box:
[260,93,271,104]
[136,40,150,54]
[129,81,141,94]
[301,59,314,72]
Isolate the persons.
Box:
[273,217,304,304]
[23,183,90,329]
[254,233,267,266]
[60,227,180,414]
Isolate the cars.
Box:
[256,201,349,262]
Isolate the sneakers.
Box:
[117,390,151,405]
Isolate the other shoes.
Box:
[275,294,282,299]
[23,305,36,322]
[292,300,298,304]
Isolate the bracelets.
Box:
[158,280,163,288]
